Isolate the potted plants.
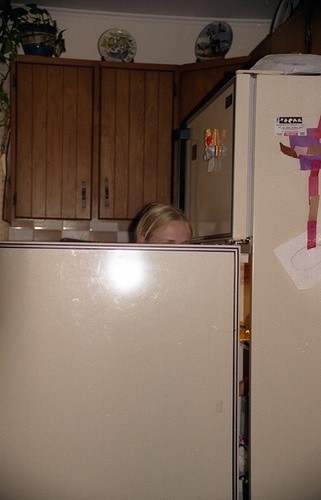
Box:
[1,2,67,152]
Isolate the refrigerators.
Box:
[0,70,321,500]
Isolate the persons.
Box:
[128,203,192,244]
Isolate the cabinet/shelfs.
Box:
[181,56,251,124]
[265,1,321,54]
[3,54,176,227]
